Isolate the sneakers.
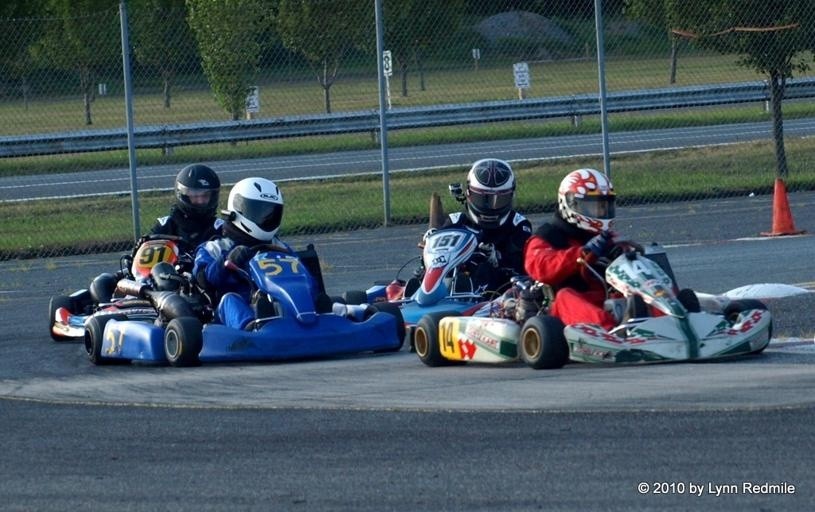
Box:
[622,294,646,324]
[679,288,700,312]
[254,298,273,319]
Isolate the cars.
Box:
[49,229,773,368]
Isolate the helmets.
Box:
[176,164,220,216]
[465,158,516,228]
[558,168,616,233]
[227,177,284,241]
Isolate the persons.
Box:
[417,159,533,306]
[193,176,334,331]
[126,164,227,293]
[523,168,678,333]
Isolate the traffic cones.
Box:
[761,179,808,236]
[418,194,445,250]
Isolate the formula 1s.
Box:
[49,229,773,368]
